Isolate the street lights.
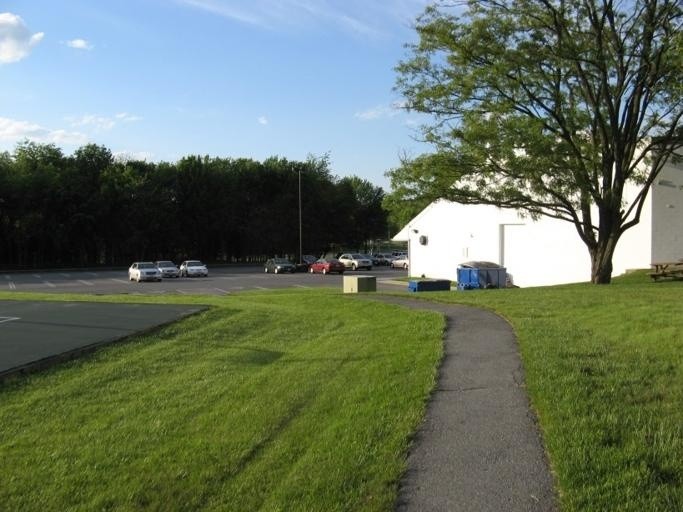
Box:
[291,166,306,264]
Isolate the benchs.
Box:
[648,268,683,282]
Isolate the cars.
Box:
[179,260,209,278]
[128,261,162,283]
[154,260,179,278]
[263,250,408,275]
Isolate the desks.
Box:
[649,261,683,273]
